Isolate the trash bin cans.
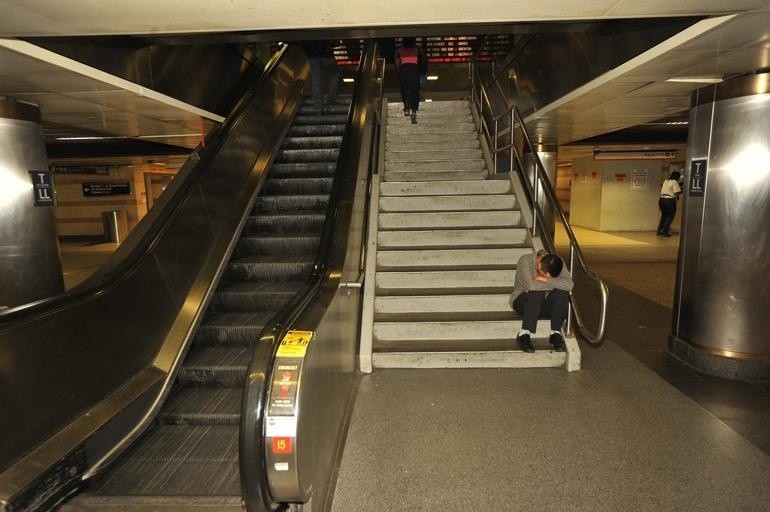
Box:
[101,210,129,243]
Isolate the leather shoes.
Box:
[517,332,534,353]
[550,332,565,351]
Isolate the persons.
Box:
[657,170,682,237]
[393,37,425,124]
[305,39,341,112]
[509,249,575,353]
